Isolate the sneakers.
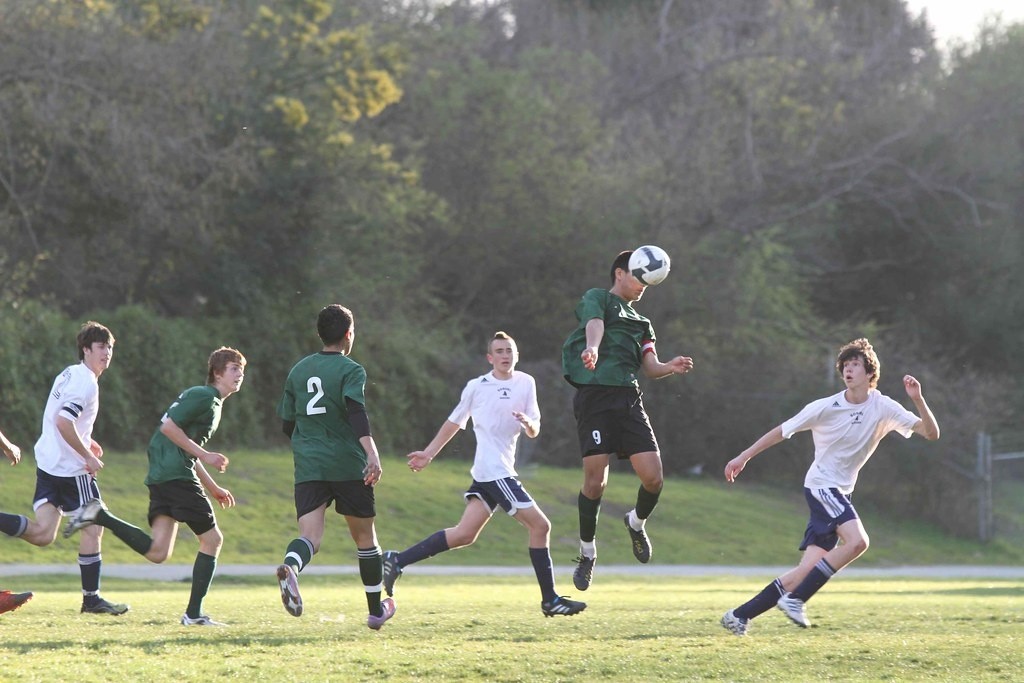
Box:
[368,598,398,630]
[382,550,403,598]
[541,596,587,618]
[624,512,652,563]
[0,590,34,615]
[180,613,226,629]
[81,598,129,616]
[571,546,598,592]
[276,564,303,617]
[778,592,812,629]
[61,498,107,539]
[720,610,751,639]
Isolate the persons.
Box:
[720,337,939,637]
[63,345,247,627]
[276,304,396,630]
[382,330,587,619]
[0,321,129,617]
[0,430,32,614]
[561,251,694,590]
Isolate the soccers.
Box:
[627,244,671,286]
